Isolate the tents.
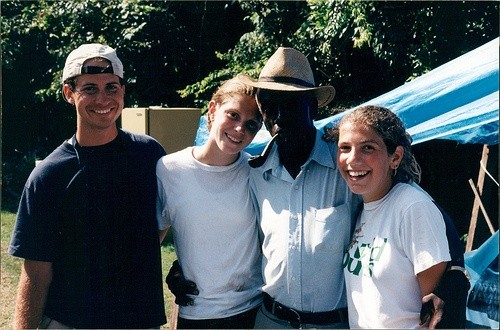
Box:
[193,33,499,270]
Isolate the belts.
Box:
[262,291,348,330]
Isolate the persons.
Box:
[166,45,474,330]
[10,42,166,330]
[157,74,340,330]
[321,105,453,330]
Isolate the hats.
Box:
[237,47,335,110]
[63,42,124,83]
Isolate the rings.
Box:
[173,272,180,278]
